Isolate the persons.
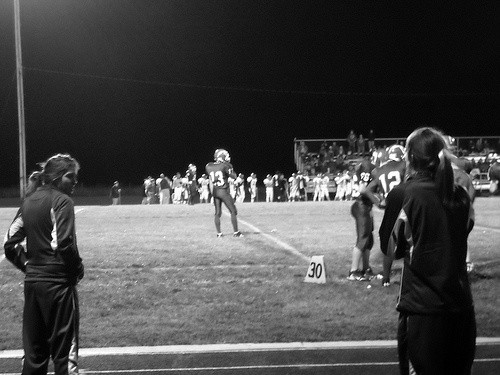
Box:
[379,126,477,375]
[4,152,84,375]
[109,181,121,205]
[347,135,476,287]
[227,171,307,203]
[142,164,209,204]
[205,149,243,239]
[297,128,500,202]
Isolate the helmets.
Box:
[385,144,406,162]
[214,149,230,162]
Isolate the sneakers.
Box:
[347,267,376,281]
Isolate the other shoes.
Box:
[382,278,390,287]
[217,233,224,238]
[233,231,243,237]
